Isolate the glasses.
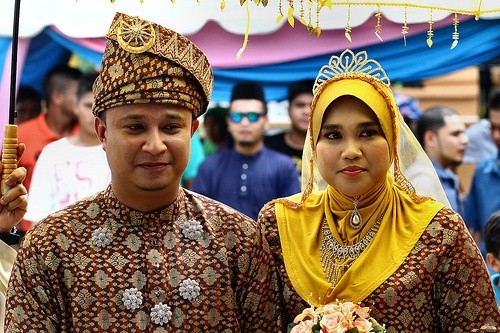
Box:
[92,13,214,117]
[228,112,265,122]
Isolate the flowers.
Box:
[285,298,388,333]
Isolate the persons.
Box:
[0,66,113,333]
[177,79,313,221]
[255,46,500,332]
[5,12,285,333]
[389,49,499,312]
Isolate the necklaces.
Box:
[319,205,388,289]
[349,194,362,229]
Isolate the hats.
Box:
[308,47,400,167]
[230,82,266,102]
[288,79,319,102]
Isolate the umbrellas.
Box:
[0,0,22,194]
